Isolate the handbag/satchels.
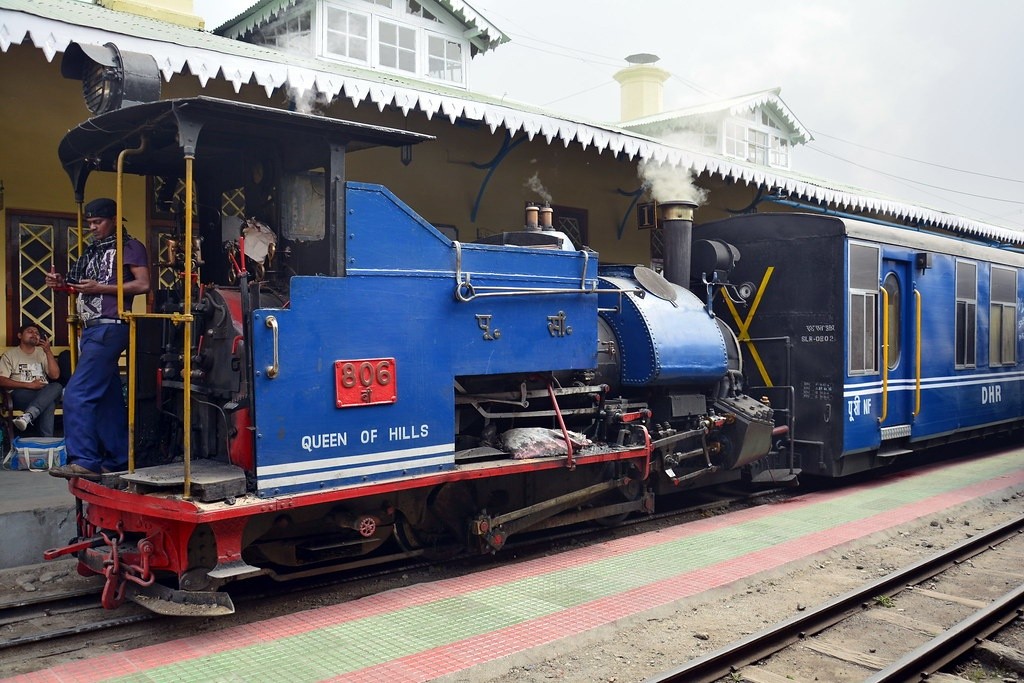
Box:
[2,435,67,472]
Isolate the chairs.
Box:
[0,355,64,446]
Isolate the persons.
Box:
[45,198,150,479]
[0,323,63,438]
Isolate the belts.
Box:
[82,318,122,328]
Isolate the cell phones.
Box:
[68,278,80,284]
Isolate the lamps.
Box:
[749,187,757,214]
[401,118,413,166]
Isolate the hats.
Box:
[84,197,127,222]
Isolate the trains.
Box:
[42,38,1023,618]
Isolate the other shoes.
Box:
[49,463,101,481]
[99,465,126,473]
[12,413,34,430]
